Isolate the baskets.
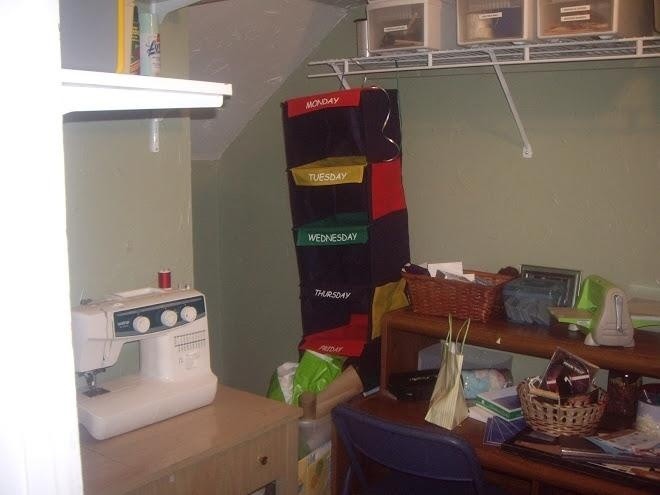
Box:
[516,376,609,439]
[400,270,523,324]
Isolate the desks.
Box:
[77,386,303,494]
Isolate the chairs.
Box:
[329,407,480,492]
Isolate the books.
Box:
[468,385,523,425]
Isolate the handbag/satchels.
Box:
[424,312,470,431]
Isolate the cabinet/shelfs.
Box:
[279,84,412,366]
[363,1,443,53]
[537,0,621,40]
[455,1,531,46]
[330,299,660,495]
